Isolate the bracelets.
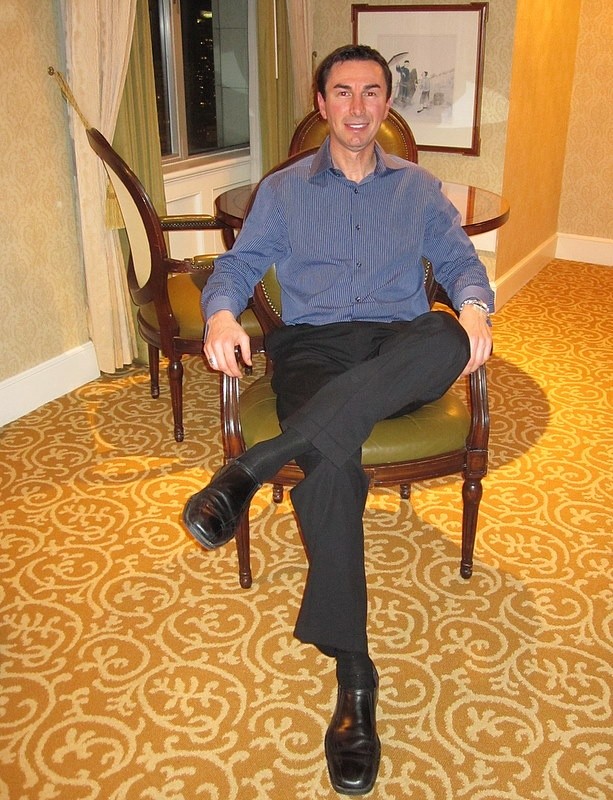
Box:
[460,300,490,320]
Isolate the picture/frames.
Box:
[351,2,489,158]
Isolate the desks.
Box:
[213,176,510,251]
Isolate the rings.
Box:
[209,357,216,365]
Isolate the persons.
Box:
[183,45,496,794]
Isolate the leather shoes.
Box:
[182,454,260,549]
[324,659,381,795]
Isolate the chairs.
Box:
[220,147,490,589]
[85,129,265,443]
[289,106,419,165]
